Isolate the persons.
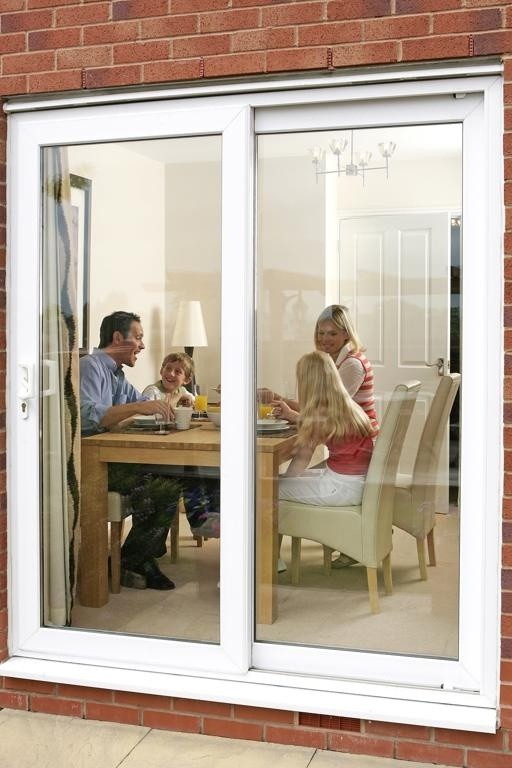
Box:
[78,310,176,592]
[270,349,373,573]
[142,351,220,539]
[257,303,380,570]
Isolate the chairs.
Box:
[107,484,206,593]
[277,374,460,614]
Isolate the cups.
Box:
[258,406,273,419]
[194,395,208,413]
[174,406,192,430]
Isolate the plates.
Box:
[133,414,172,425]
[255,419,291,433]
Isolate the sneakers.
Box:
[330,558,360,569]
[190,516,220,538]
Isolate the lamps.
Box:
[308,130,397,187]
[169,297,207,398]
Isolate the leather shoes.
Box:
[108,553,176,591]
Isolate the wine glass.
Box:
[154,393,171,434]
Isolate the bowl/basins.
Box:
[207,412,221,428]
